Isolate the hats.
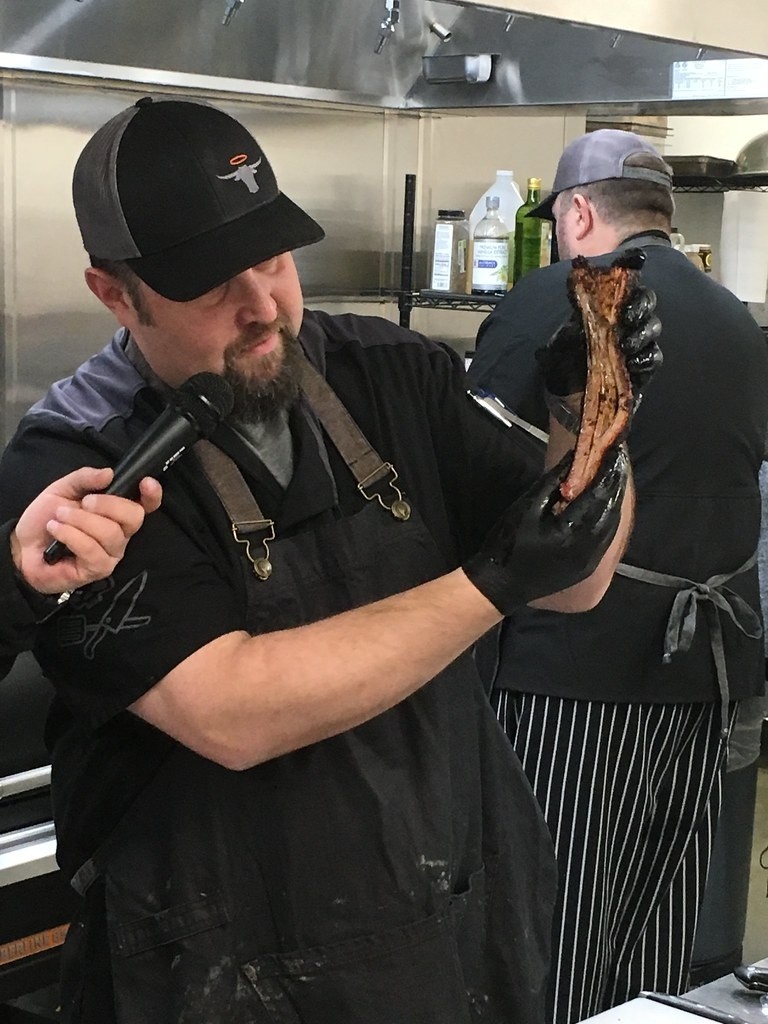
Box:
[524,129,674,219]
[72,97,325,302]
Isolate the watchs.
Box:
[13,566,76,605]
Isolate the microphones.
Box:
[41,371,233,565]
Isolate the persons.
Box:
[0,466,163,680]
[0,98,663,1024]
[462,128,768,1024]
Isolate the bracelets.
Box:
[539,390,580,434]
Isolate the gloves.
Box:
[533,246,664,395]
[461,441,628,620]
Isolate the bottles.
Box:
[470,170,524,242]
[669,228,685,256]
[426,210,470,293]
[472,197,510,294]
[514,178,553,288]
[684,244,713,279]
